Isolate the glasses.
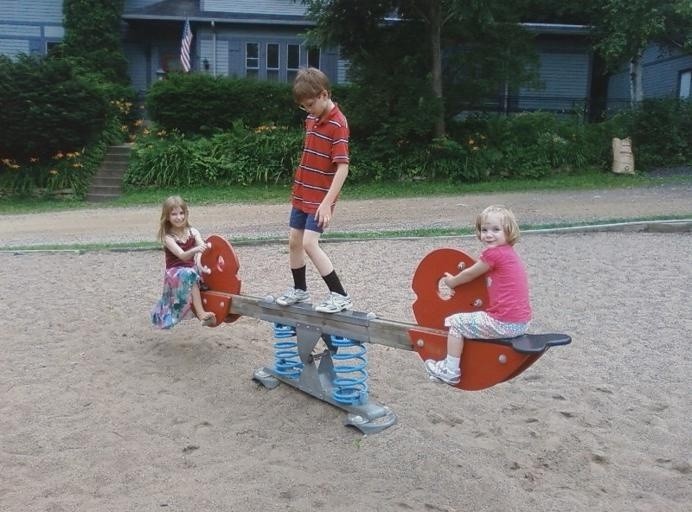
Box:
[299,96,321,112]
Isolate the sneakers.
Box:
[200,311,217,326]
[424,358,461,386]
[315,290,354,314]
[275,288,312,307]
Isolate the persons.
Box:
[425,202,532,386]
[149,194,218,330]
[275,68,357,313]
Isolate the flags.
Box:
[179,17,193,74]
